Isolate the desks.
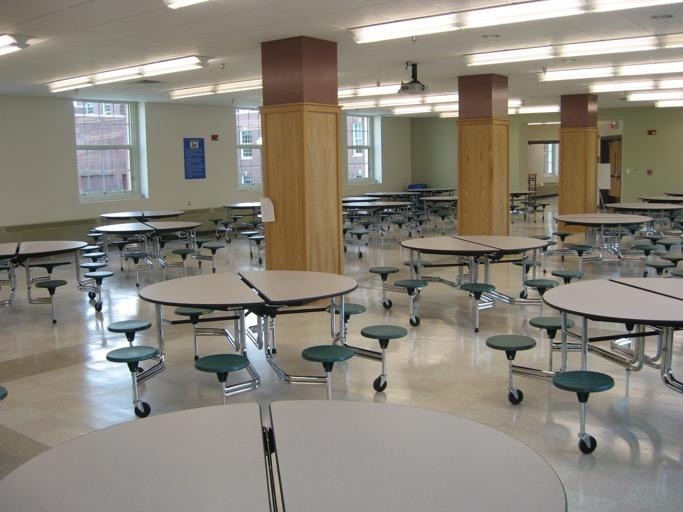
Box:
[3,400,567,510]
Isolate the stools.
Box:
[340,189,562,260]
[369,194,683,332]
[107,270,408,418]
[0,187,268,325]
[486,278,683,454]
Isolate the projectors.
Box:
[397,80,426,93]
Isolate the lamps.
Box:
[654,101,683,108]
[340,95,459,109]
[627,92,681,102]
[589,80,680,93]
[540,62,681,82]
[439,106,560,117]
[466,35,681,69]
[349,1,681,45]
[171,80,265,100]
[337,85,408,98]
[41,55,215,94]
[394,100,522,114]
[2,32,46,59]
